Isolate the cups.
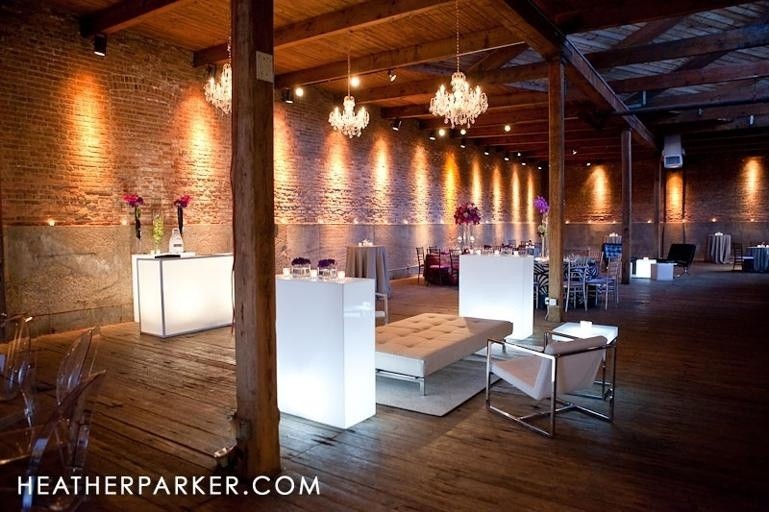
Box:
[756,244,769,248]
[357,242,373,247]
[469,249,518,255]
[606,235,622,244]
[337,270,345,281]
[715,232,723,237]
[282,266,290,276]
[309,269,318,279]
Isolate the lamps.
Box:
[90,32,106,57]
[202,0,511,161]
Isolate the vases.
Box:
[541,216,549,258]
[135,207,142,253]
[318,268,337,278]
[291,266,310,277]
[178,207,185,238]
[463,222,471,248]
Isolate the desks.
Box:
[706,234,731,264]
[345,246,390,298]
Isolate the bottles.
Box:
[167,228,189,255]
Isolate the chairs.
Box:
[375,292,389,325]
[746,247,769,272]
[0,313,108,512]
[416,240,622,312]
[733,241,754,272]
[485,330,617,440]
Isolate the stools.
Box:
[656,262,673,281]
[375,313,513,396]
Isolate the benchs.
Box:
[656,244,696,279]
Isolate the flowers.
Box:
[318,259,336,267]
[174,195,190,236]
[291,257,311,266]
[454,202,481,246]
[534,195,550,256]
[124,194,145,240]
